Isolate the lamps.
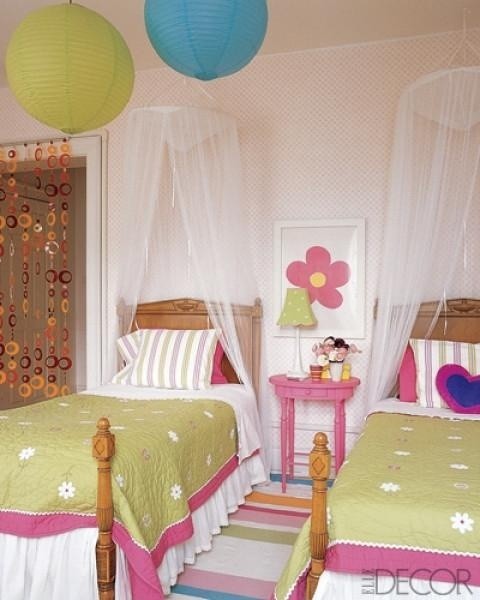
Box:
[276,284,319,382]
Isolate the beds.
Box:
[271,297,480,600]
[2,297,269,599]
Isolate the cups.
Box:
[309,365,323,380]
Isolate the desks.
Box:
[268,373,361,494]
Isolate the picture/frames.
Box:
[274,218,369,342]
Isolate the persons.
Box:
[315,336,356,378]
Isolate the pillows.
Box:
[108,327,222,391]
[398,347,416,402]
[210,341,230,385]
[408,333,480,416]
